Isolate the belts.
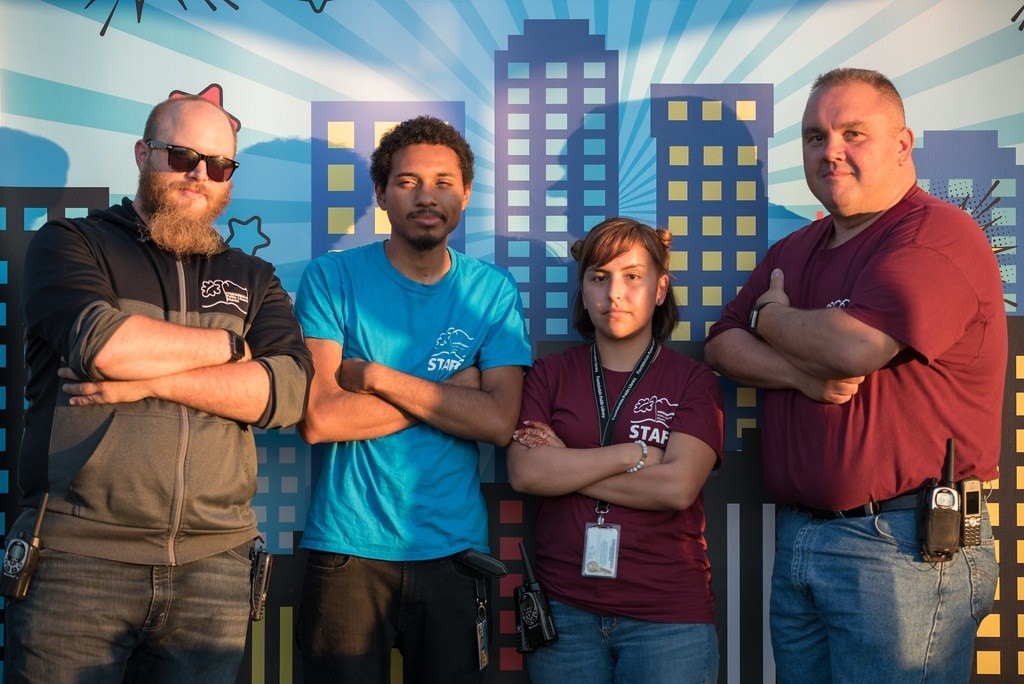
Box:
[785,494,918,520]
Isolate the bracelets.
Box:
[627,440,648,473]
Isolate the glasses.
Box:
[147,139,239,182]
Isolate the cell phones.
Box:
[251,545,272,622]
[960,479,982,547]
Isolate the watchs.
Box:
[749,302,773,337]
[223,328,245,363]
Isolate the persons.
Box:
[0,98,316,684]
[704,68,1009,684]
[507,218,723,683]
[295,116,530,684]
